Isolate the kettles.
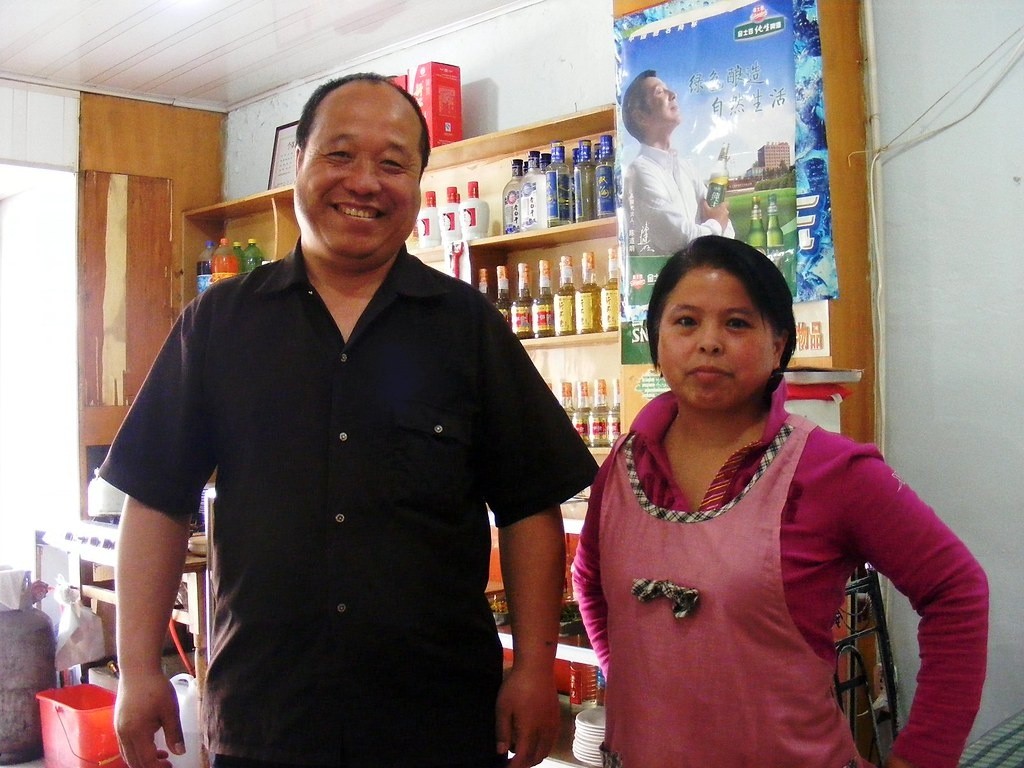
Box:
[86,464,126,517]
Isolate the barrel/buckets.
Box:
[34,684,128,768]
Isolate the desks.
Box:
[68,550,212,767]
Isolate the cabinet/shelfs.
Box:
[177,100,623,768]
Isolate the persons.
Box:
[571,236,993,767]
[623,71,739,252]
[97,70,600,768]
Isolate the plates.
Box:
[572,707,606,767]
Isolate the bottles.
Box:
[154,673,200,768]
[570,633,605,706]
[196,238,266,293]
[579,252,602,334]
[545,379,621,448]
[767,194,783,246]
[707,143,730,207]
[458,194,460,204]
[438,186,460,244]
[515,263,534,339]
[460,181,490,241]
[495,266,512,329]
[536,259,555,337]
[418,191,441,248]
[477,269,492,303]
[603,248,620,332]
[747,196,766,248]
[557,256,576,336]
[501,135,617,234]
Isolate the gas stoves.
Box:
[42,516,203,571]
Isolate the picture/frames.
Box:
[268,120,300,190]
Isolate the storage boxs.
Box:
[406,61,464,149]
[388,74,408,94]
